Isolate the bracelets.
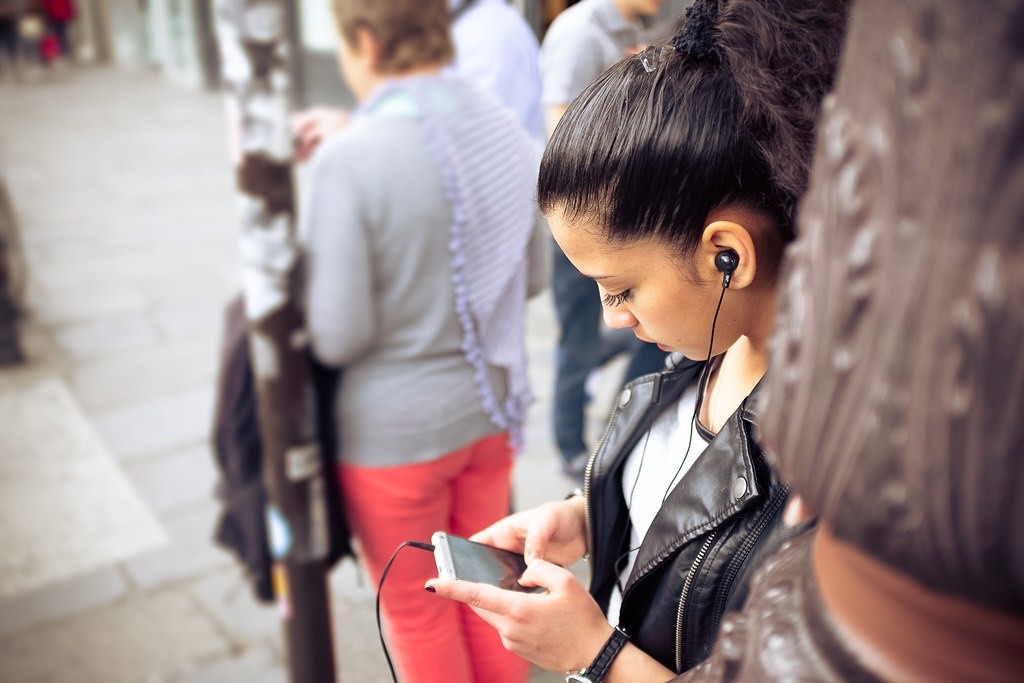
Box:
[564,486,592,561]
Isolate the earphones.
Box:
[715,250,739,288]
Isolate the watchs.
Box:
[563,625,630,683]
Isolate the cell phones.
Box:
[431,530,563,594]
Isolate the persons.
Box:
[283,2,556,682]
[419,0,820,683]
[445,1,547,150]
[539,0,670,477]
[219,0,303,89]
